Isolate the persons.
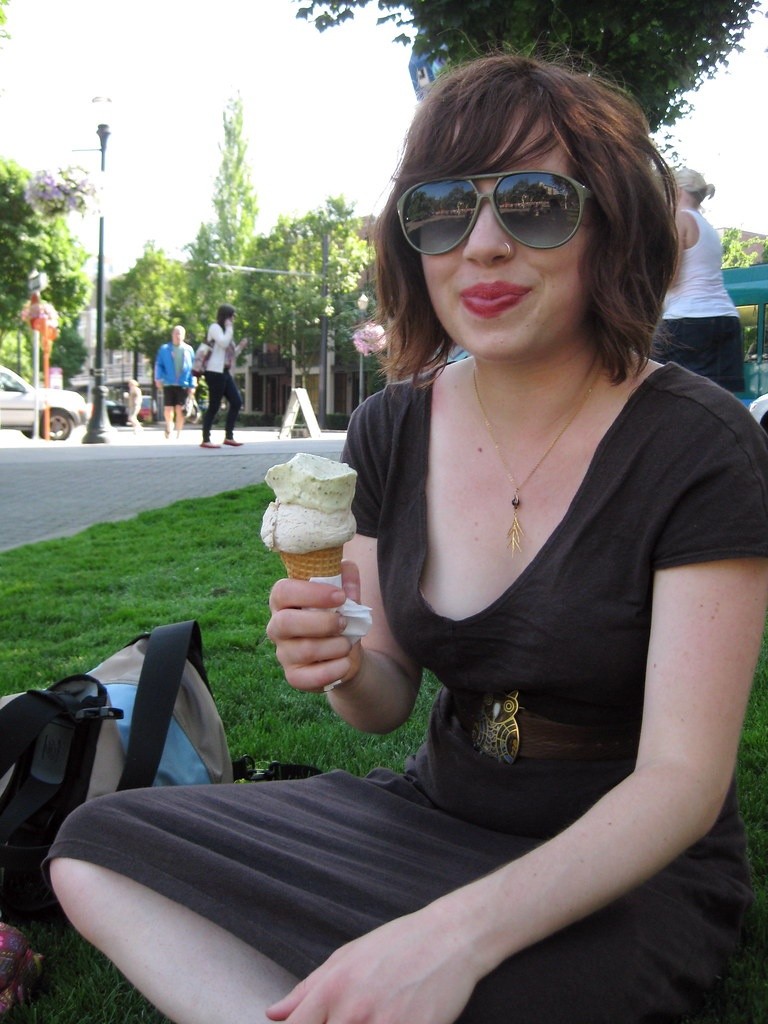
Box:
[128,379,144,436]
[199,306,247,448]
[154,325,198,438]
[40,55,768,1024]
[651,169,746,393]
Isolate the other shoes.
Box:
[224,439,243,446]
[200,443,220,449]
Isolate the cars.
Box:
[0,365,87,441]
[104,399,128,426]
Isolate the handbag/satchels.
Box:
[192,342,215,377]
[0,619,231,918]
[184,397,201,423]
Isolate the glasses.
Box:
[396,170,597,256]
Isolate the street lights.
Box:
[357,292,370,406]
[83,94,116,444]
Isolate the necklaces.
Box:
[473,357,604,558]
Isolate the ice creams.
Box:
[260,452,358,581]
